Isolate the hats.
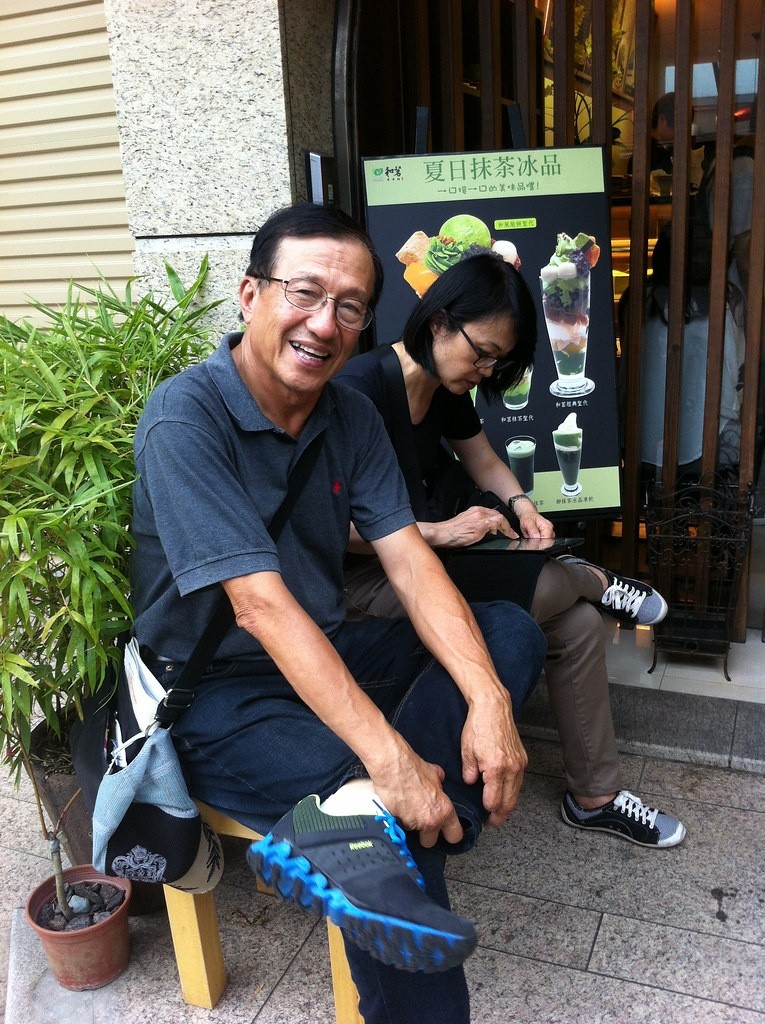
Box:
[91,729,224,895]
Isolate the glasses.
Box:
[457,324,513,371]
[252,277,374,332]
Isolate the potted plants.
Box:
[0,257,231,991]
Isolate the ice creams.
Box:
[554,411,583,451]
[439,214,491,252]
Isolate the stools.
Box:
[158,800,366,1024]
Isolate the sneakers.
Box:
[561,790,686,847]
[558,555,668,625]
[246,796,479,972]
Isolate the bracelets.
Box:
[507,495,538,511]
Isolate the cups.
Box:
[539,272,596,398]
[498,363,533,410]
[552,427,584,501]
[504,435,536,495]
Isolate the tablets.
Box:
[451,536,585,558]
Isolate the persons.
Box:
[700,93,757,414]
[614,216,746,503]
[132,202,552,1024]
[342,251,687,847]
[627,92,699,179]
